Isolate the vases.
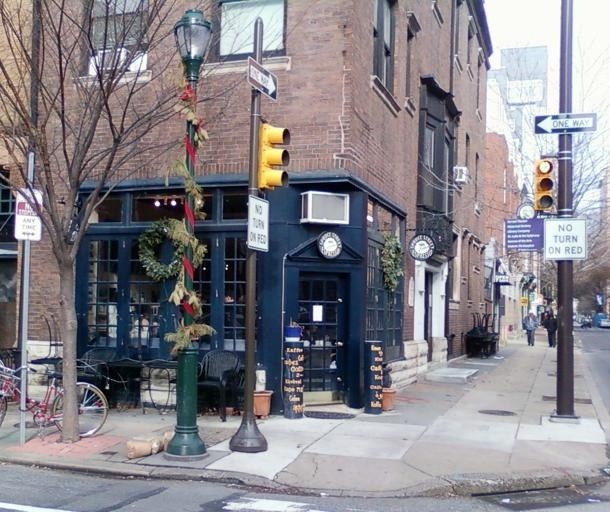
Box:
[381,389,397,412]
[251,390,273,419]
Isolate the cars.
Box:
[572,312,610,329]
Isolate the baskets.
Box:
[0,370,21,397]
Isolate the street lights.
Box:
[161,3,216,465]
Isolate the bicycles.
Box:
[1,357,111,441]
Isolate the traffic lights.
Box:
[533,155,556,213]
[257,119,292,196]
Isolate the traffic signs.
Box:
[530,112,599,136]
[246,53,280,104]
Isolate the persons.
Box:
[523,309,538,345]
[543,314,558,348]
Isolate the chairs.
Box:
[31,313,246,425]
[465,310,502,360]
